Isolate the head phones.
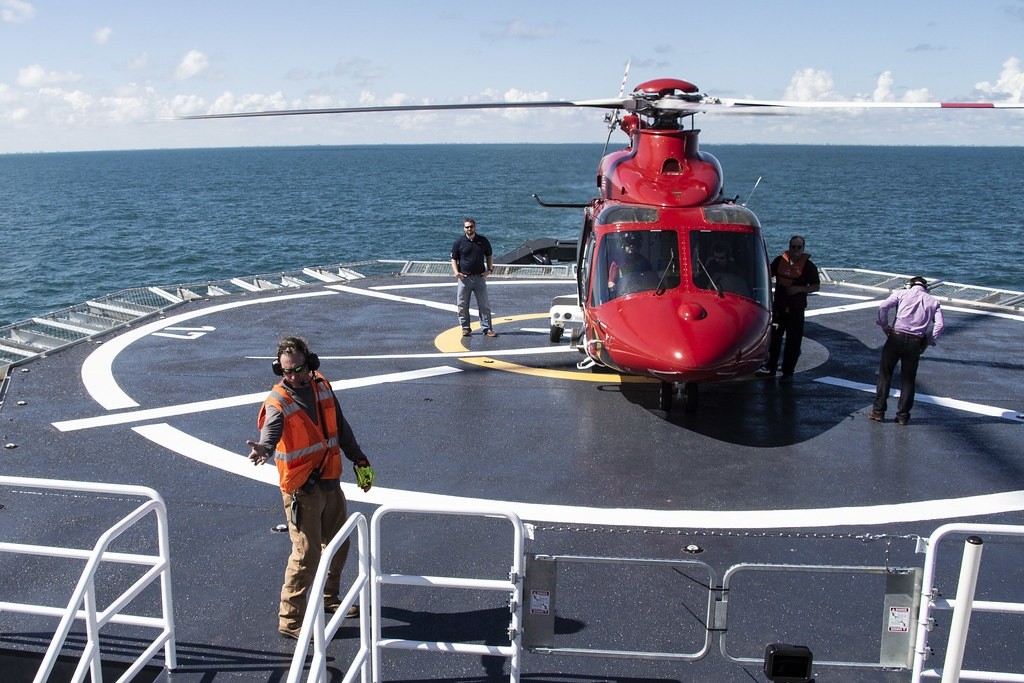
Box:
[272,348,320,376]
[904,281,932,293]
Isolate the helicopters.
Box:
[181,60,1024,422]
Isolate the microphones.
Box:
[300,371,315,386]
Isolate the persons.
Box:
[704,245,746,290]
[768,236,820,384]
[449,219,497,337]
[245,338,374,644]
[867,276,945,425]
[608,231,652,288]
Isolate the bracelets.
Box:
[488,269,493,274]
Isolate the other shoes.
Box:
[897,415,907,424]
[868,412,883,420]
[462,328,471,335]
[483,329,496,337]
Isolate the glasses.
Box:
[280,359,307,374]
[791,244,802,249]
[465,224,475,229]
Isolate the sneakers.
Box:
[324,603,358,618]
[754,367,776,376]
[278,625,314,643]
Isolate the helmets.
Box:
[619,231,641,247]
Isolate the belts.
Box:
[891,330,915,338]
